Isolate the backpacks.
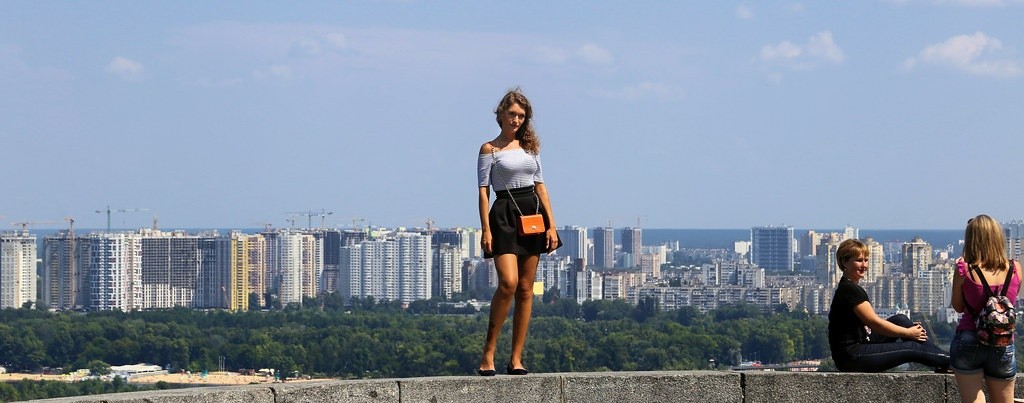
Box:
[963,261,1016,347]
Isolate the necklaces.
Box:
[496,140,501,151]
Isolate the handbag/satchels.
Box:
[517,214,546,236]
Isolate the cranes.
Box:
[94,205,152,232]
[288,210,320,229]
[11,221,63,231]
[341,217,365,227]
[64,216,76,309]
[317,208,333,230]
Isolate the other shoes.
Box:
[934,366,953,373]
[479,369,496,376]
[507,363,528,375]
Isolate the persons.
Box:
[477,92,563,375]
[949,215,1023,403]
[827,239,951,373]
